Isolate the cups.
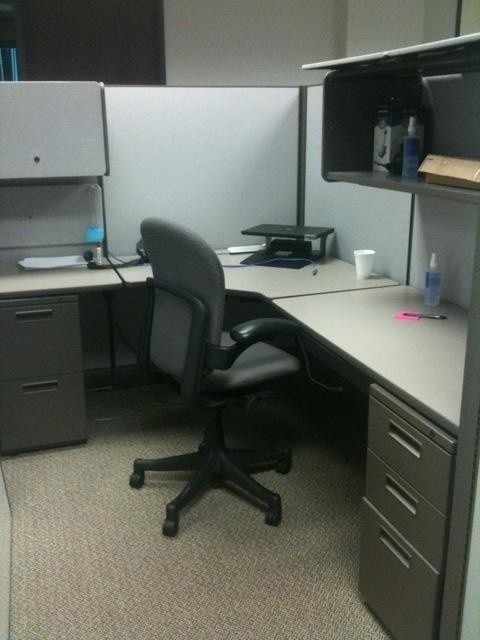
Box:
[353,249,376,280]
[0,47,18,81]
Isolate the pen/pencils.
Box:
[404,313,447,319]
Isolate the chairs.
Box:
[127,216,303,537]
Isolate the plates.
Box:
[17,255,81,269]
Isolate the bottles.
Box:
[424,251,441,306]
[96,245,103,264]
[402,115,420,177]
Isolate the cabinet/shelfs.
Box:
[355,377,459,640]
[0,295,88,456]
[301,28,480,206]
